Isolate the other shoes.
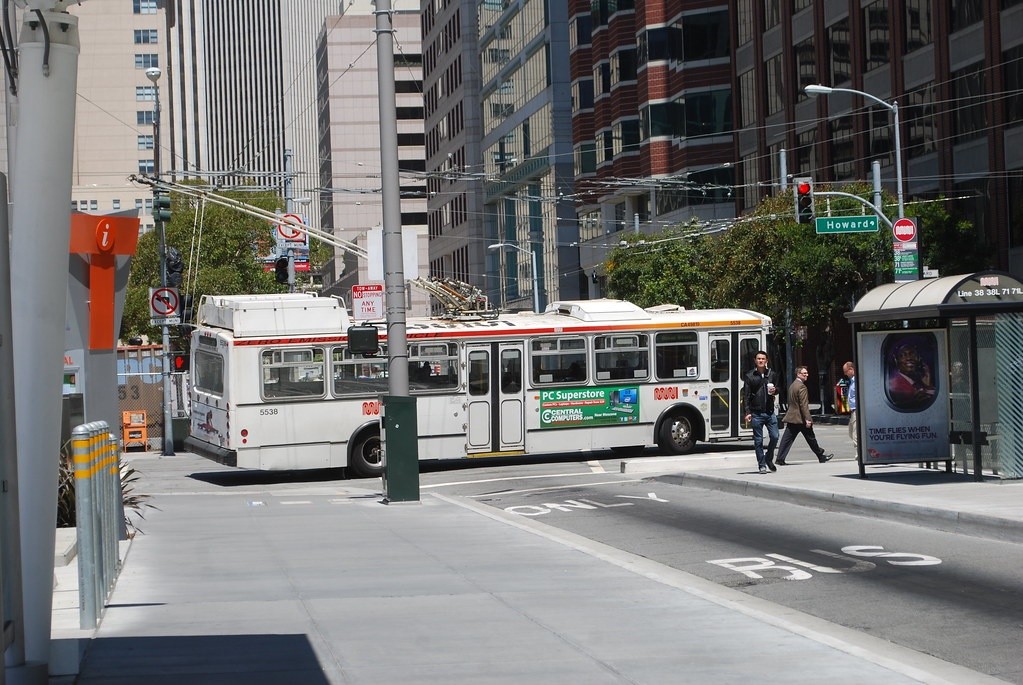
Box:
[765,456,776,471]
[759,466,767,474]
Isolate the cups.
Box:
[767,382,774,395]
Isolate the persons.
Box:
[951,362,968,394]
[775,365,834,465]
[843,362,858,461]
[743,351,780,474]
[890,342,931,400]
[421,361,431,377]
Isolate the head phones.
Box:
[892,343,921,369]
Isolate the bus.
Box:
[186,294,771,478]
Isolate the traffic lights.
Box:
[152,193,171,223]
[275,257,289,284]
[793,178,815,225]
[178,293,194,336]
[174,355,189,372]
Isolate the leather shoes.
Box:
[775,461,789,465]
[820,454,834,463]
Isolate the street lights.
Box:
[145,67,175,456]
[488,243,539,315]
[286,196,313,293]
[806,85,904,220]
